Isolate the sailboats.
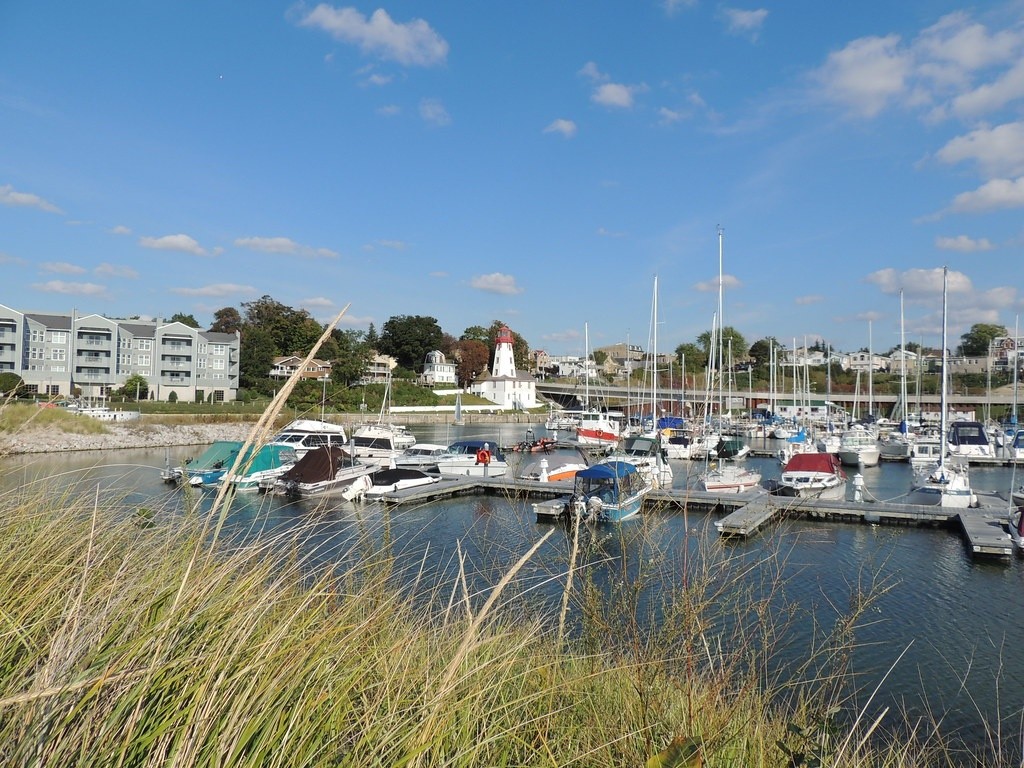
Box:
[157,226,1024,510]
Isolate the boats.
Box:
[1006,506,1024,547]
[572,462,654,523]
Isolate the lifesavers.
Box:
[478,450,490,464]
[596,429,603,438]
